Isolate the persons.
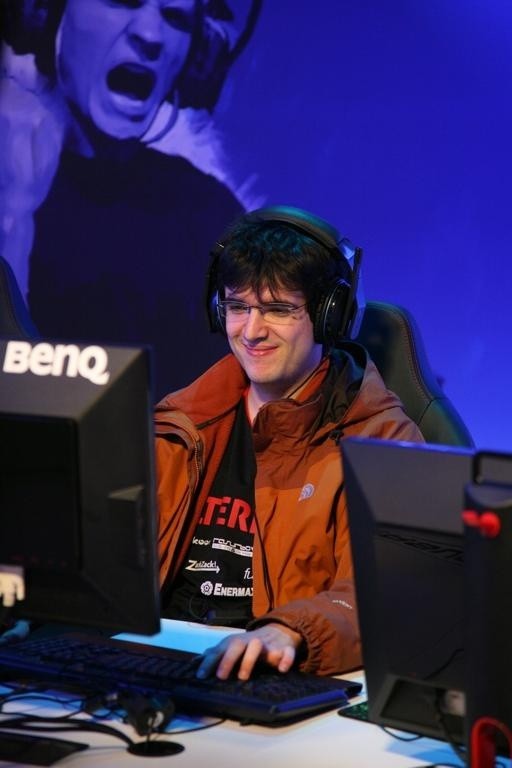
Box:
[153,204,428,682]
[0,0,247,408]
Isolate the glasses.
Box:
[217,298,309,319]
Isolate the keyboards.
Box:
[0,635,362,724]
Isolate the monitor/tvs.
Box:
[342,440,512,768]
[1,339,161,767]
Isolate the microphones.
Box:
[112,87,179,159]
[342,245,363,336]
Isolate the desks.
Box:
[0,617,511,766]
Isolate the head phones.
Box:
[205,205,358,343]
[0,0,263,111]
